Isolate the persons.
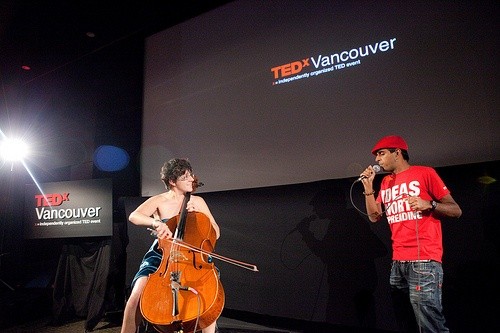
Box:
[120,158,220,333]
[360,135,462,333]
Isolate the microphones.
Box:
[355,165,381,182]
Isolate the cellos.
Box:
[140,176,225,333]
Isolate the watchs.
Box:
[429,200,438,212]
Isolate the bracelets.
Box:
[362,190,375,196]
[151,219,163,229]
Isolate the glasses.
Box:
[175,173,195,181]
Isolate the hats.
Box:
[371,135,408,156]
